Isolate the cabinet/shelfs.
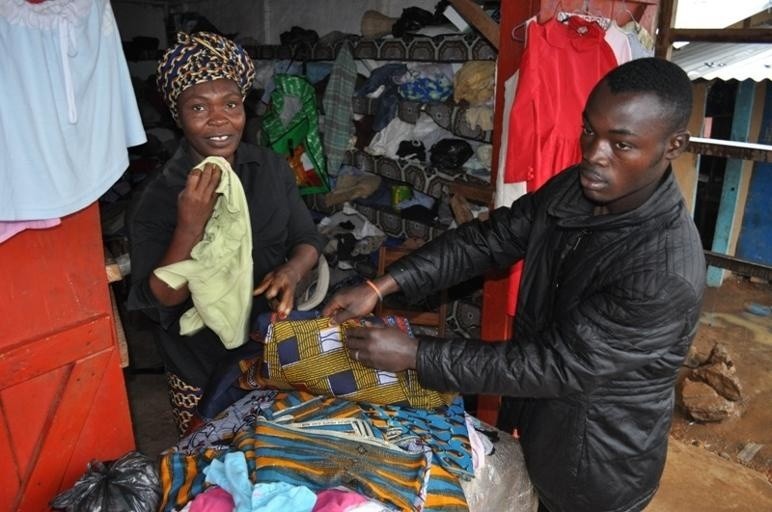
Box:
[97,0,503,430]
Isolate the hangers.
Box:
[512,0,663,45]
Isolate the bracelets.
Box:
[364,278,382,302]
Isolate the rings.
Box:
[355,351,359,361]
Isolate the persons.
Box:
[126,32,323,444]
[321,57,706,512]
